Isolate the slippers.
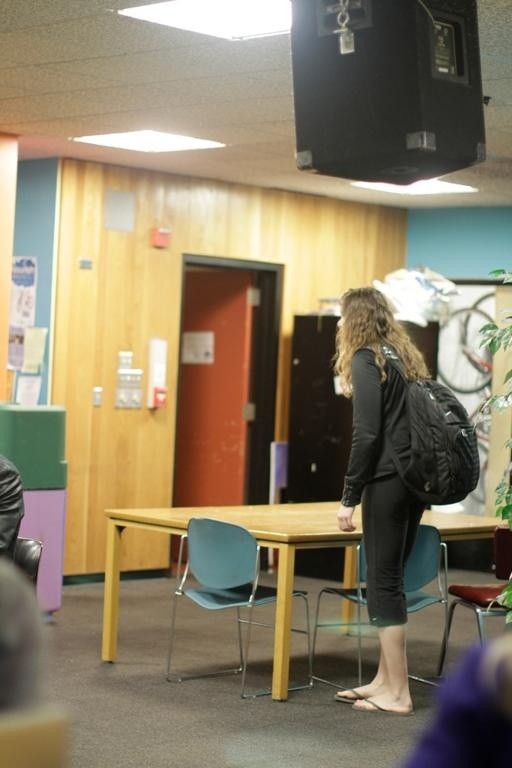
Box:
[332,688,415,717]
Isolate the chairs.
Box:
[311,524,451,689]
[166,518,315,699]
[440,581,512,677]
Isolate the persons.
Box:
[331,287,433,716]
[0,454,25,562]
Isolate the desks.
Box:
[98,500,511,703]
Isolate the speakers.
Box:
[290,0,487,186]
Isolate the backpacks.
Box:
[351,341,480,505]
